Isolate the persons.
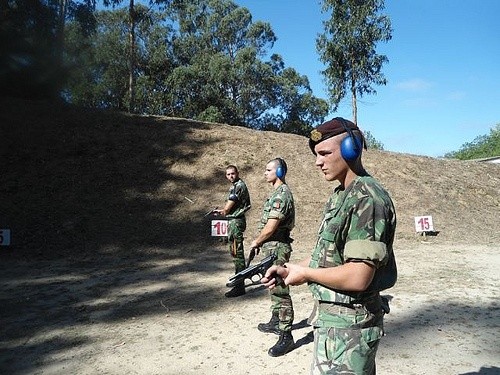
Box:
[248,158,301,358]
[214,165,248,299]
[260,117,396,375]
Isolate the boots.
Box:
[269,330,294,356]
[258,312,281,335]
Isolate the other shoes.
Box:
[226,288,245,297]
[226,281,235,287]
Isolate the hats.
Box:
[309,117,359,156]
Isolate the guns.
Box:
[203,207,222,218]
[227,254,286,289]
